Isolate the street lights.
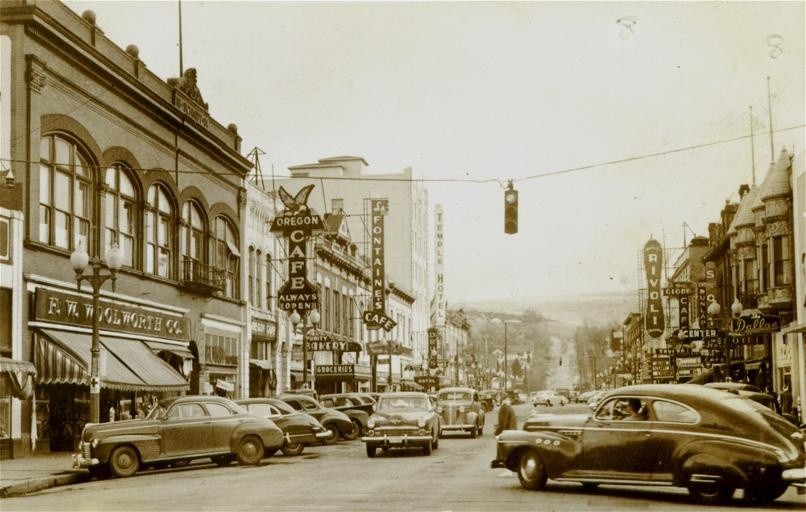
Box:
[663,328,685,383]
[448,358,524,394]
[290,307,321,389]
[707,296,744,382]
[70,235,124,424]
[419,348,437,392]
[596,340,661,390]
[380,334,399,391]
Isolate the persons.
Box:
[490,392,517,461]
[411,400,422,409]
[460,394,469,402]
[623,399,646,422]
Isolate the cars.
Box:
[700,381,806,431]
[490,383,806,506]
[71,395,291,477]
[231,397,333,456]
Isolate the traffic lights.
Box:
[504,190,518,235]
